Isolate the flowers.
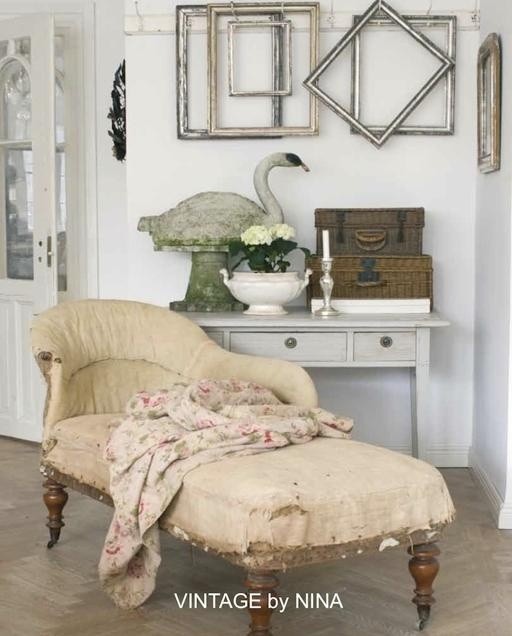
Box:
[239,224,298,273]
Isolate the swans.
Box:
[137,151,310,244]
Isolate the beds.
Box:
[32,296,457,636]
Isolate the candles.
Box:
[323,228,330,259]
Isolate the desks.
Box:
[178,306,451,460]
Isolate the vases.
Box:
[220,269,314,317]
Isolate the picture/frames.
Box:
[175,1,456,151]
[476,32,502,175]
